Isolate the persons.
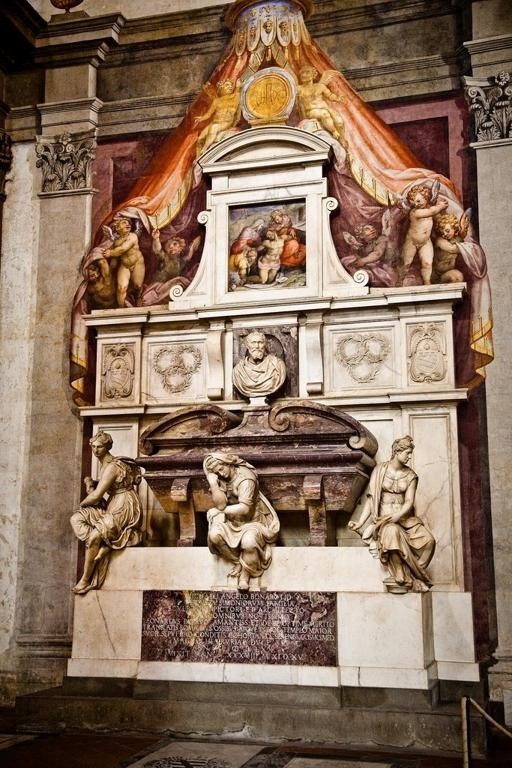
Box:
[193,77,244,160]
[68,428,145,593]
[294,66,350,151]
[232,332,286,397]
[201,451,280,591]
[343,183,464,287]
[346,434,436,595]
[83,217,200,309]
[228,209,306,284]
